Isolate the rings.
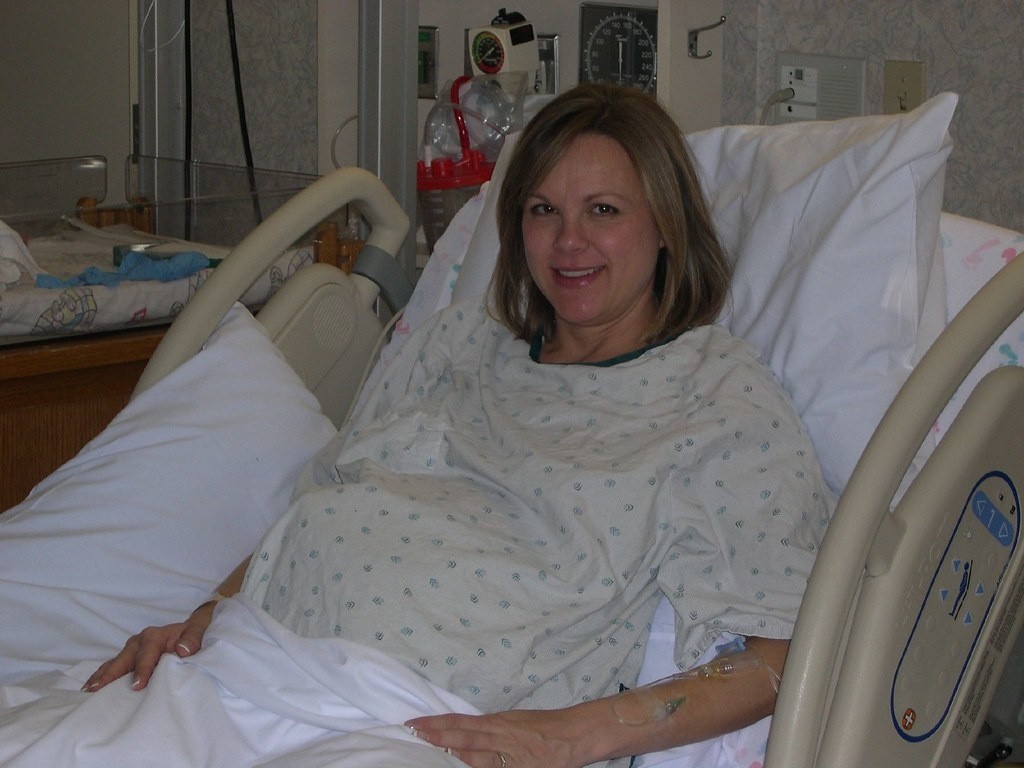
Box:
[497,752,505,768]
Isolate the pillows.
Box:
[454,88,963,521]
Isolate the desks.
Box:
[0,326,169,513]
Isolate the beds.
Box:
[0,170,1024,768]
[0,152,328,347]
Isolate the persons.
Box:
[80,81,838,768]
